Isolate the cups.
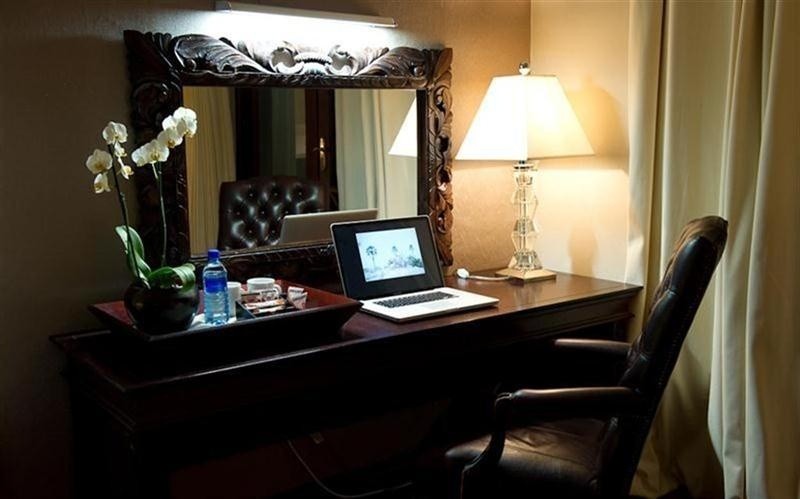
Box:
[246,277,282,291]
[225,281,244,317]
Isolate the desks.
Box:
[47,266,643,497]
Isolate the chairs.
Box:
[415,217,728,498]
[217,176,330,251]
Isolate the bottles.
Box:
[202,247,229,324]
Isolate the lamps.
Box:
[215,2,398,28]
[388,96,417,157]
[455,61,597,286]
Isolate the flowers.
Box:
[86,107,198,291]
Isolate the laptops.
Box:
[279,209,378,243]
[330,215,499,323]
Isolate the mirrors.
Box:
[183,86,418,255]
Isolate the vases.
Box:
[123,283,200,335]
